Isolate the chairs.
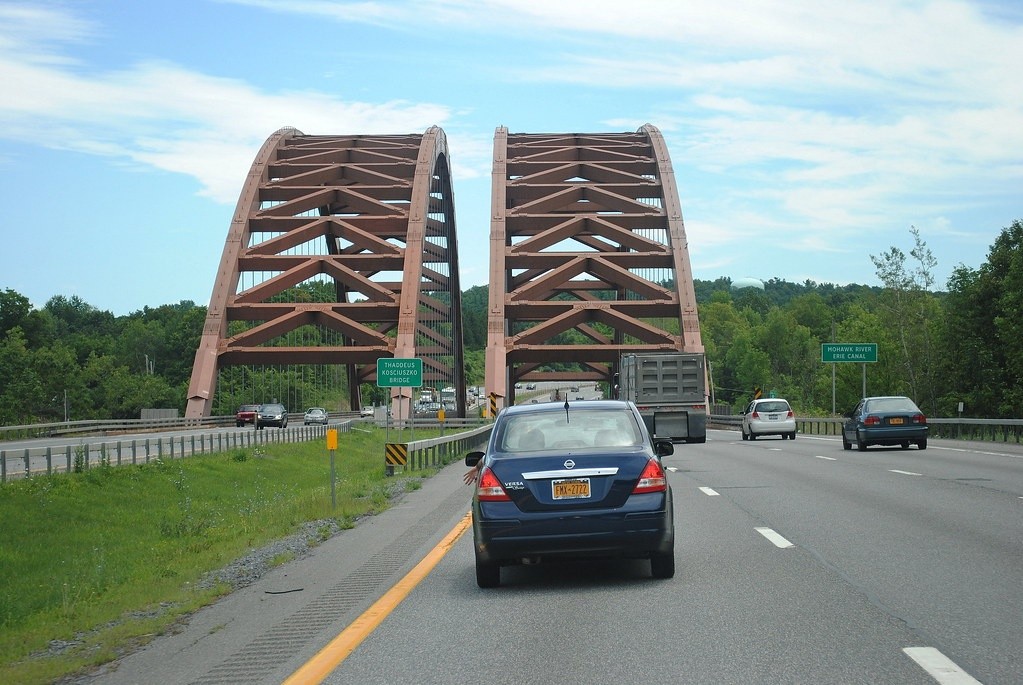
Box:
[518,429,546,449]
[594,428,623,447]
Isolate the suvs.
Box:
[236,405,262,428]
[256,403,287,430]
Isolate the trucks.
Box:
[442,388,456,403]
[613,352,707,445]
[419,387,437,404]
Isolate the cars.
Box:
[576,395,584,400]
[842,396,928,452]
[570,384,579,392]
[380,405,391,418]
[304,407,328,425]
[594,382,604,391]
[360,405,373,418]
[526,383,537,390]
[465,398,675,588]
[412,384,482,415]
[739,399,796,441]
[514,383,523,389]
